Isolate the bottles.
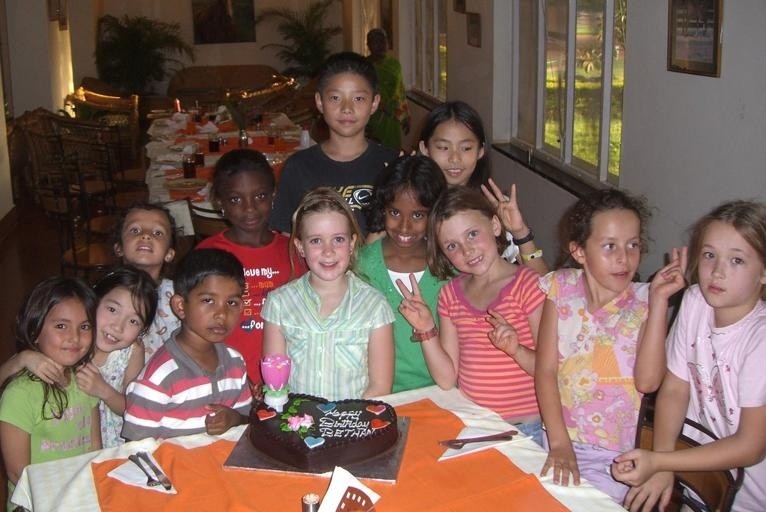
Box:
[239,129,248,147]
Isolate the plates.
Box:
[190,201,224,219]
[163,179,207,191]
[293,144,312,151]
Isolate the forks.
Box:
[128,455,160,487]
[438,436,512,449]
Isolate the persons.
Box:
[531,188,688,505]
[266,51,397,250]
[611,200,766,512]
[0,273,104,511]
[118,247,256,442]
[363,27,411,151]
[111,197,181,363]
[394,186,550,453]
[348,152,552,397]
[0,260,159,448]
[261,184,396,404]
[410,100,511,267]
[194,149,310,385]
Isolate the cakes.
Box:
[249,354,396,472]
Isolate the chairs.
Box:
[631,394,749,511]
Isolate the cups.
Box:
[207,103,217,121]
[192,143,204,167]
[267,133,276,146]
[192,107,201,122]
[183,153,197,179]
[300,130,310,145]
[207,133,219,152]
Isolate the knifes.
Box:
[439,430,517,443]
[135,451,172,492]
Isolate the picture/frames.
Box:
[453,0,467,14]
[466,11,482,48]
[665,0,724,77]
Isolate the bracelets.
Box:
[513,231,535,245]
[408,324,441,344]
[520,250,545,260]
